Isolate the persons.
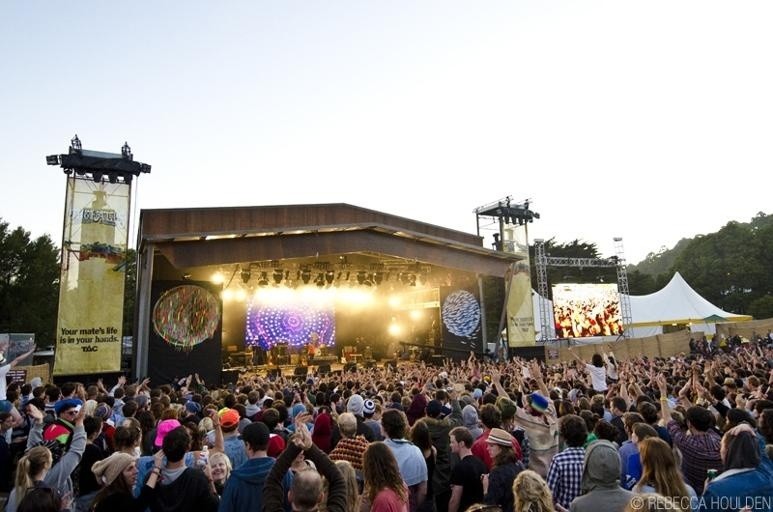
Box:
[0,330,773,512]
[550,290,621,337]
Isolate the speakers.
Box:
[432,355,446,365]
[293,367,307,375]
[317,365,329,373]
[438,276,510,365]
[384,360,396,367]
[363,360,376,368]
[267,369,281,377]
[149,280,223,388]
[222,369,239,385]
[344,362,356,372]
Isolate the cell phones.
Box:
[707,469,718,482]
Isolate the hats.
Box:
[526,392,548,413]
[485,428,513,447]
[347,393,376,419]
[238,421,269,439]
[154,419,182,447]
[722,377,735,386]
[218,407,241,428]
[93,402,111,422]
[91,451,136,487]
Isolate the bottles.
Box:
[199,445,208,466]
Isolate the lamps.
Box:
[241,255,427,287]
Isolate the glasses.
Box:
[69,411,78,415]
